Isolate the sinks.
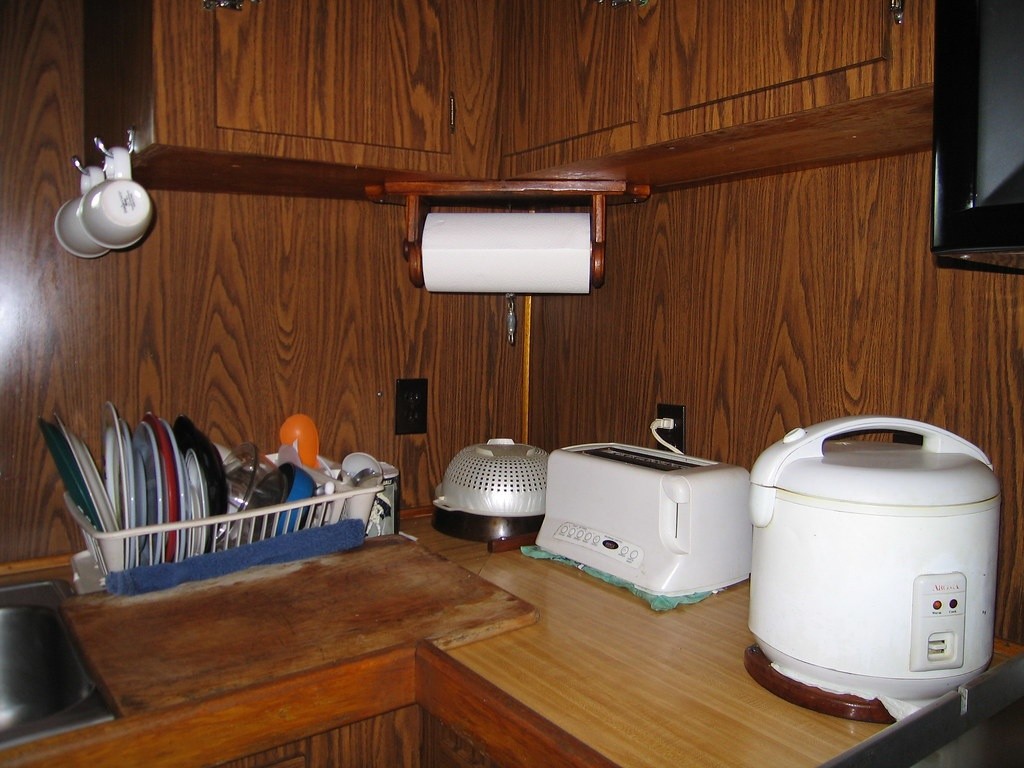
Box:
[0,579,121,747]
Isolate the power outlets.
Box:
[395,379,428,434]
[657,404,686,455]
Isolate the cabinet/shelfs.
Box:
[503,0,934,196]
[82,0,503,203]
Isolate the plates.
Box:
[37,401,229,569]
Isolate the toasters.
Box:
[536,441,752,593]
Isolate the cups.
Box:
[77,148,152,249]
[339,453,382,487]
[53,167,108,259]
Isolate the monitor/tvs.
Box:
[932,0,1024,253]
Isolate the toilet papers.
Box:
[420,209,594,297]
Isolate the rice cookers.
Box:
[745,415,1000,700]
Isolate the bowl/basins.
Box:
[216,443,316,545]
